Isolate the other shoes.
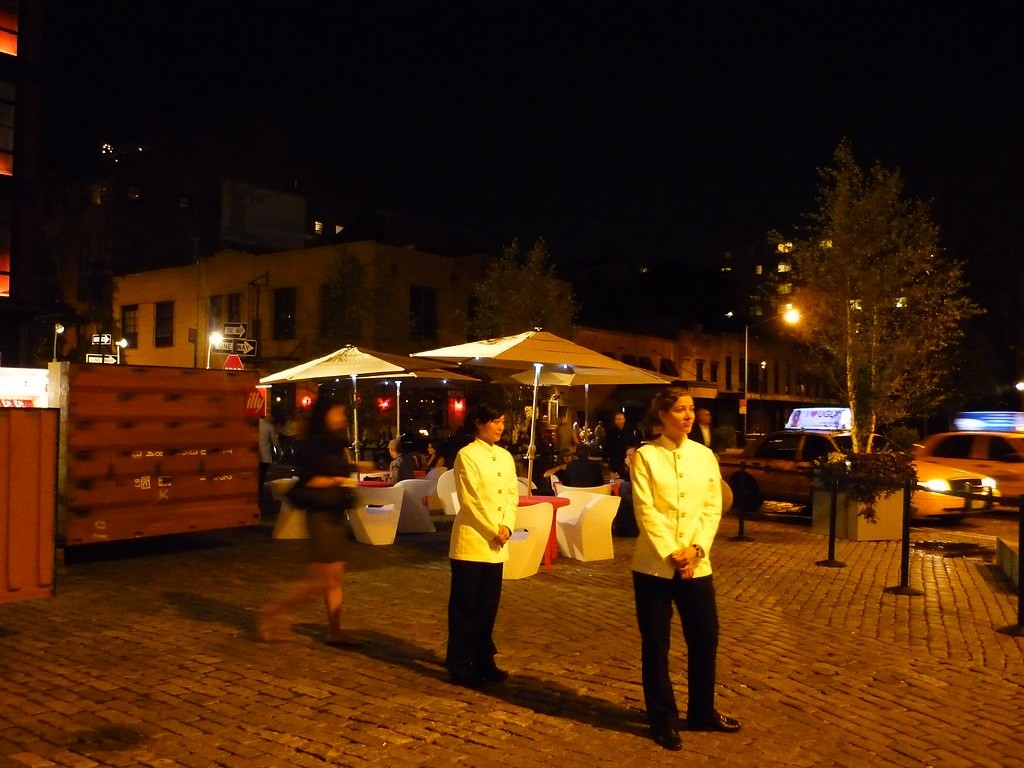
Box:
[474,656,509,680]
[446,657,485,682]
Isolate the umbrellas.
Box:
[258,346,460,480]
[349,364,481,441]
[410,328,671,499]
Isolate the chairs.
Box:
[272,477,309,540]
[502,502,554,580]
[349,487,405,547]
[551,475,621,561]
[426,467,446,509]
[438,468,462,515]
[395,479,438,535]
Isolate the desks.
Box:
[358,480,393,487]
[519,495,569,569]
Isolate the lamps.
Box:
[54,323,65,361]
[114,338,128,363]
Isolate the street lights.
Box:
[737,301,801,536]
[116,339,128,364]
[54,324,64,362]
[206,332,223,370]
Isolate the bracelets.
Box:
[693,545,704,562]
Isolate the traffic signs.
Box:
[91,334,111,346]
[86,354,118,364]
[212,337,258,355]
[223,323,249,339]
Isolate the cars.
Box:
[716,406,1002,522]
[912,410,1024,515]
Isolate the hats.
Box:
[387,439,401,449]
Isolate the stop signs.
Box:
[223,354,245,370]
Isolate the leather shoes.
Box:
[649,723,682,750]
[688,708,740,732]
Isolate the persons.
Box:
[562,445,604,488]
[258,419,284,498]
[628,386,740,750]
[447,402,520,690]
[381,440,414,483]
[689,409,722,455]
[594,412,637,459]
[607,448,640,535]
[424,445,445,473]
